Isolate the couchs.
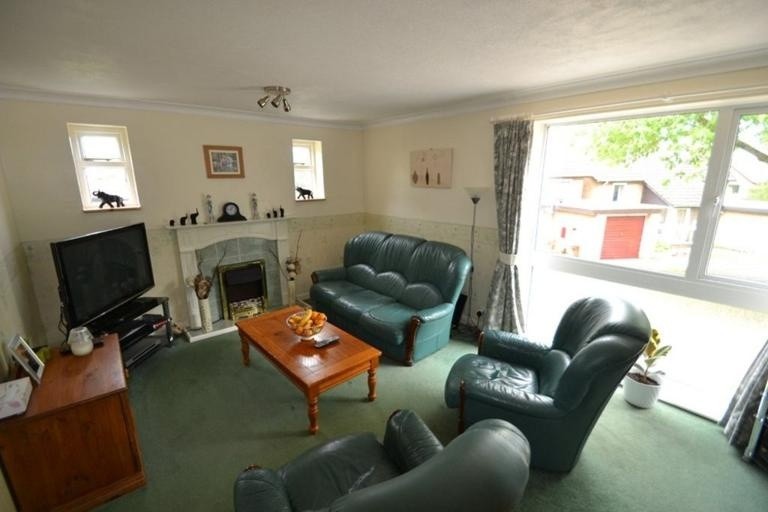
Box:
[307,231,474,367]
[231,407,532,511]
[443,295,652,478]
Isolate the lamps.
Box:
[461,185,491,334]
[255,86,292,113]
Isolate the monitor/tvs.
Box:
[50,222,155,330]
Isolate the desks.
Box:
[83,297,175,380]
[0,332,147,511]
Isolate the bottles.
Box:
[67,326,96,356]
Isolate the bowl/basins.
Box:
[285,311,329,342]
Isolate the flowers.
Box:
[268,227,305,281]
[185,239,228,299]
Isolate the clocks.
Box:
[216,201,248,222]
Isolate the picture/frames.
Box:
[8,332,47,386]
[202,145,246,179]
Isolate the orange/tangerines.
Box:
[288,309,325,336]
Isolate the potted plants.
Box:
[623,329,673,410]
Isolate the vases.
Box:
[287,280,297,307]
[197,298,214,335]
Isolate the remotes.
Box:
[313,336,340,348]
[59,336,104,355]
